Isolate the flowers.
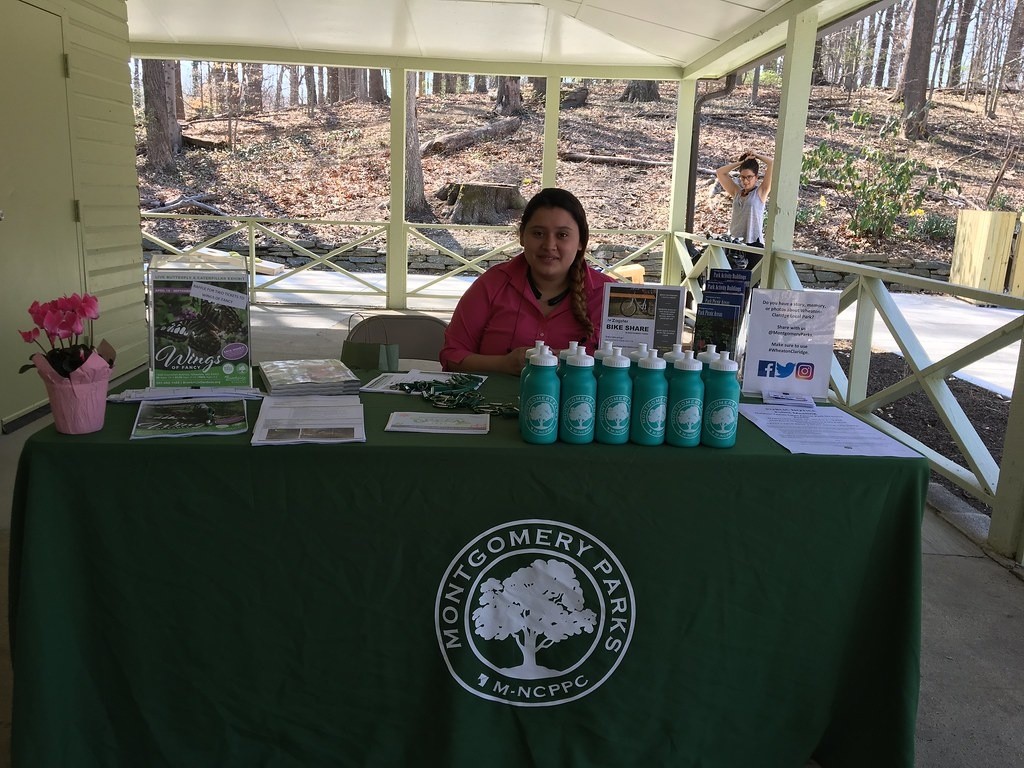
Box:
[18,293,100,378]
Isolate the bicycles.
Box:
[620,288,656,318]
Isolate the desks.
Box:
[7,366,925,768]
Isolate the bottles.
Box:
[596,347,633,444]
[555,341,587,379]
[520,345,560,443]
[701,351,740,447]
[628,343,648,382]
[663,344,685,391]
[558,347,597,444]
[631,349,668,445]
[594,341,614,379]
[697,345,721,384]
[666,350,705,446]
[521,341,553,393]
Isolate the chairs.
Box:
[346,315,449,371]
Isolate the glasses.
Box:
[740,175,756,180]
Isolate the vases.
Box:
[19,337,118,434]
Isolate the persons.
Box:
[439,188,619,376]
[717,151,772,314]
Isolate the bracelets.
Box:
[744,159,746,163]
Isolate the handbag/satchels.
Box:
[341,312,398,372]
